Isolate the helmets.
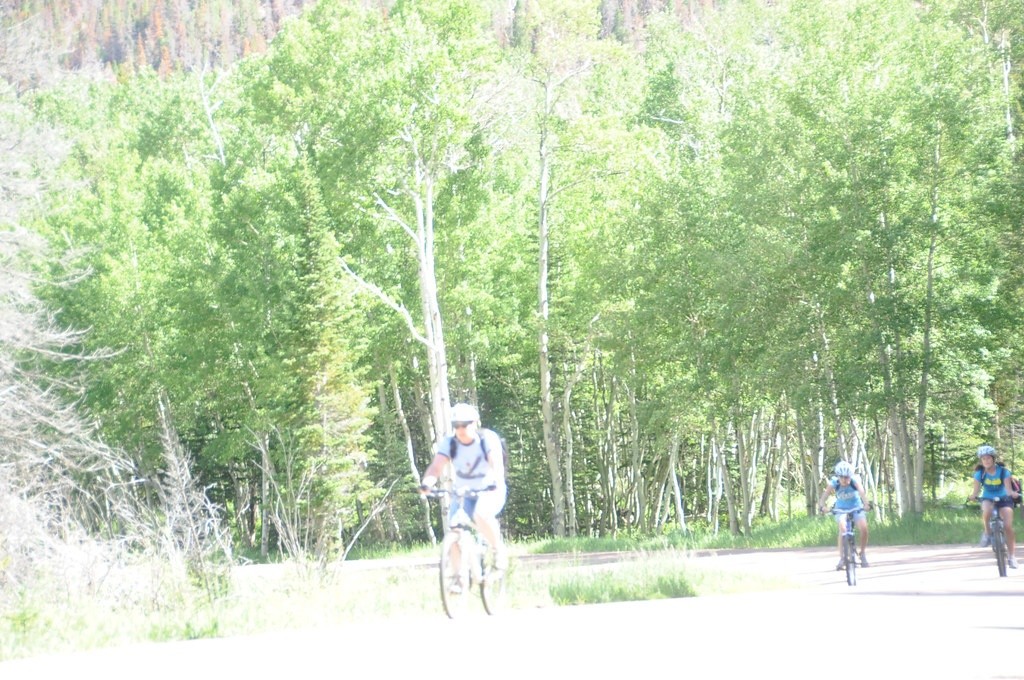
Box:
[977,446,995,458]
[834,462,854,476]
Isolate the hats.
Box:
[449,403,480,421]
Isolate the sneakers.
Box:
[836,559,845,570]
[860,553,868,567]
[981,532,989,546]
[448,574,463,594]
[487,546,509,571]
[1008,557,1017,568]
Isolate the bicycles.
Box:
[973,494,1016,576]
[829,505,866,586]
[415,483,511,619]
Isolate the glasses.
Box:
[453,424,467,428]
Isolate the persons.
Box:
[969,446,1019,569]
[419,404,507,593]
[820,461,870,570]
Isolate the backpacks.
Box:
[500,438,509,466]
[1011,476,1022,494]
